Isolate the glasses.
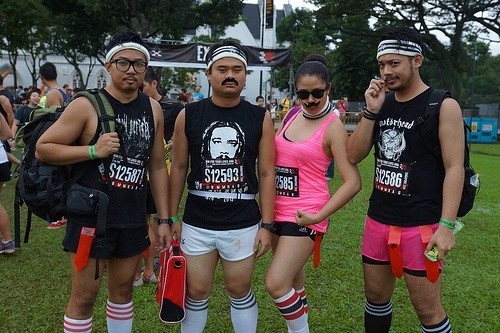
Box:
[296,86,328,100]
[111,59,148,73]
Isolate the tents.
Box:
[138,40,298,142]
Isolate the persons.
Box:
[168,38,276,333]
[257,55,362,333]
[0,60,193,289]
[346,28,465,333]
[35,33,172,333]
[177,84,350,132]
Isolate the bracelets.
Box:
[169,215,179,223]
[88,145,96,159]
[439,218,456,229]
[360,107,379,121]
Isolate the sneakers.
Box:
[0,239,15,254]
[47,216,67,229]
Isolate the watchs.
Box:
[261,221,276,235]
[158,217,173,227]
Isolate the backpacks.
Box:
[373,88,481,217]
[14,89,116,281]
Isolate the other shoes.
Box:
[141,272,157,283]
[133,277,144,287]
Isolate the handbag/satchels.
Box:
[156,237,186,324]
